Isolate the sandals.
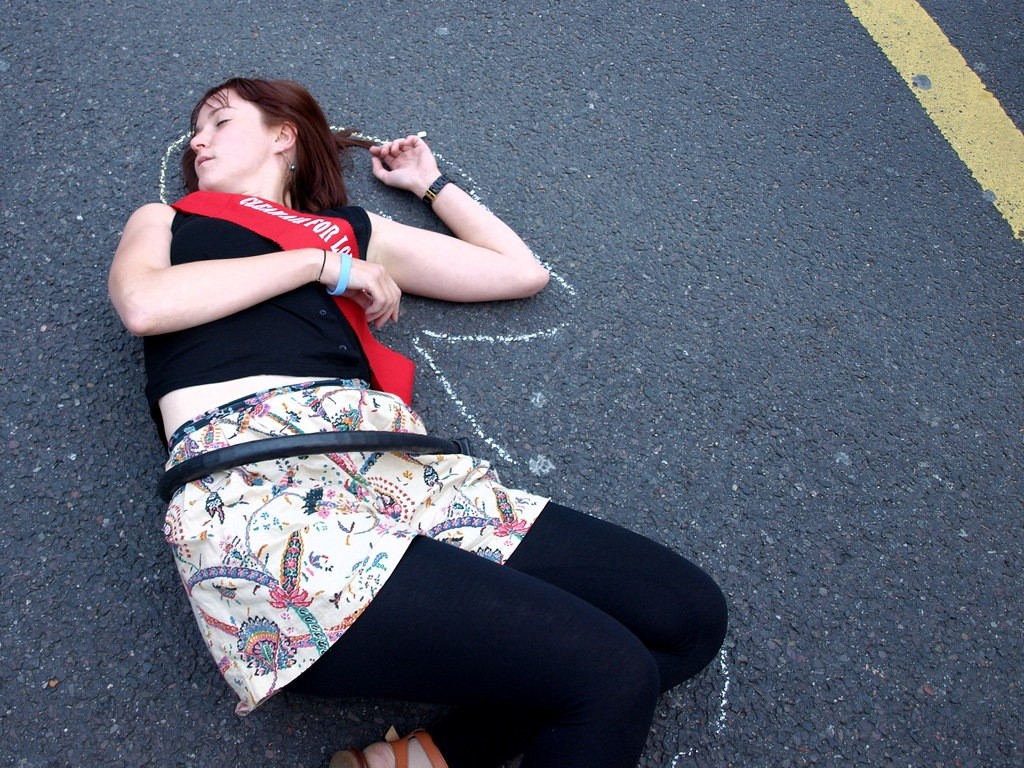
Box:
[328,725,449,768]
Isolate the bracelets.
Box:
[316,250,327,284]
[422,173,453,208]
[324,252,352,296]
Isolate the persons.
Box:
[109,76,728,768]
